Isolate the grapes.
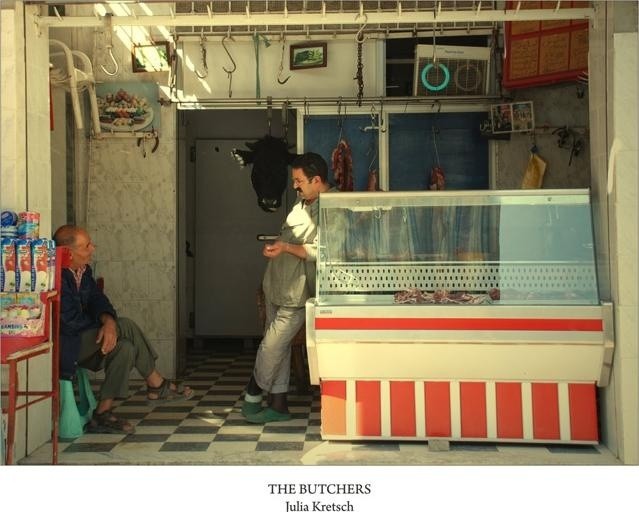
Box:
[96,88,129,112]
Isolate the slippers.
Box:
[244,393,292,424]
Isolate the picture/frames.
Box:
[130,41,171,74]
[289,41,328,70]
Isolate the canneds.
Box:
[16,211,40,241]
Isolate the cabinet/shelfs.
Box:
[1,245,65,466]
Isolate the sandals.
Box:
[89,410,135,434]
[147,377,195,404]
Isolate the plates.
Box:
[100,106,153,131]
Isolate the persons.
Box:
[241,153,350,423]
[53,225,194,435]
